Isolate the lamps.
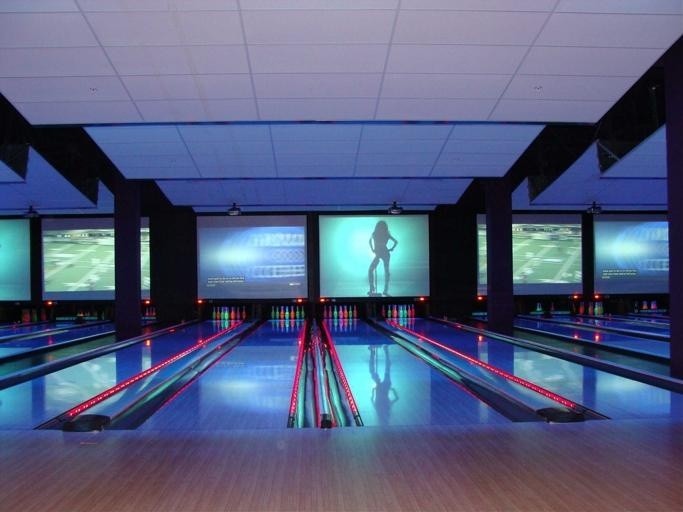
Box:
[386,199,404,217]
[584,199,605,218]
[225,200,243,218]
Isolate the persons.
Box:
[368,220,398,296]
[368,343,398,424]
